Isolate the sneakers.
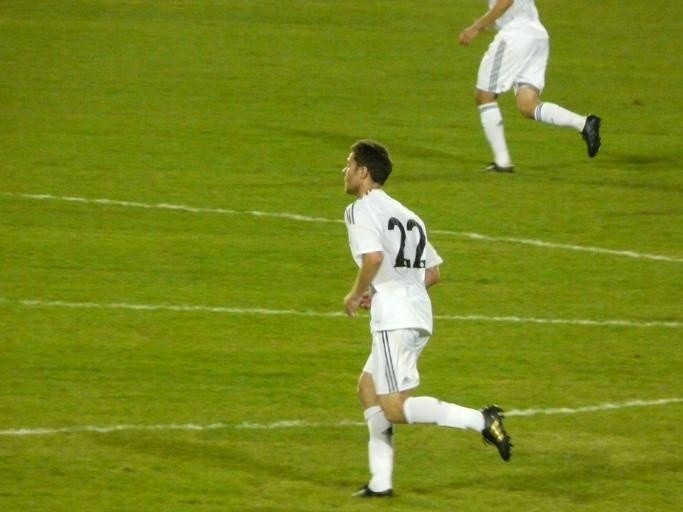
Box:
[480,405,514,461]
[352,483,394,498]
[579,115,600,158]
[479,160,516,174]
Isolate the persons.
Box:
[456,0,602,175]
[338,138,513,501]
